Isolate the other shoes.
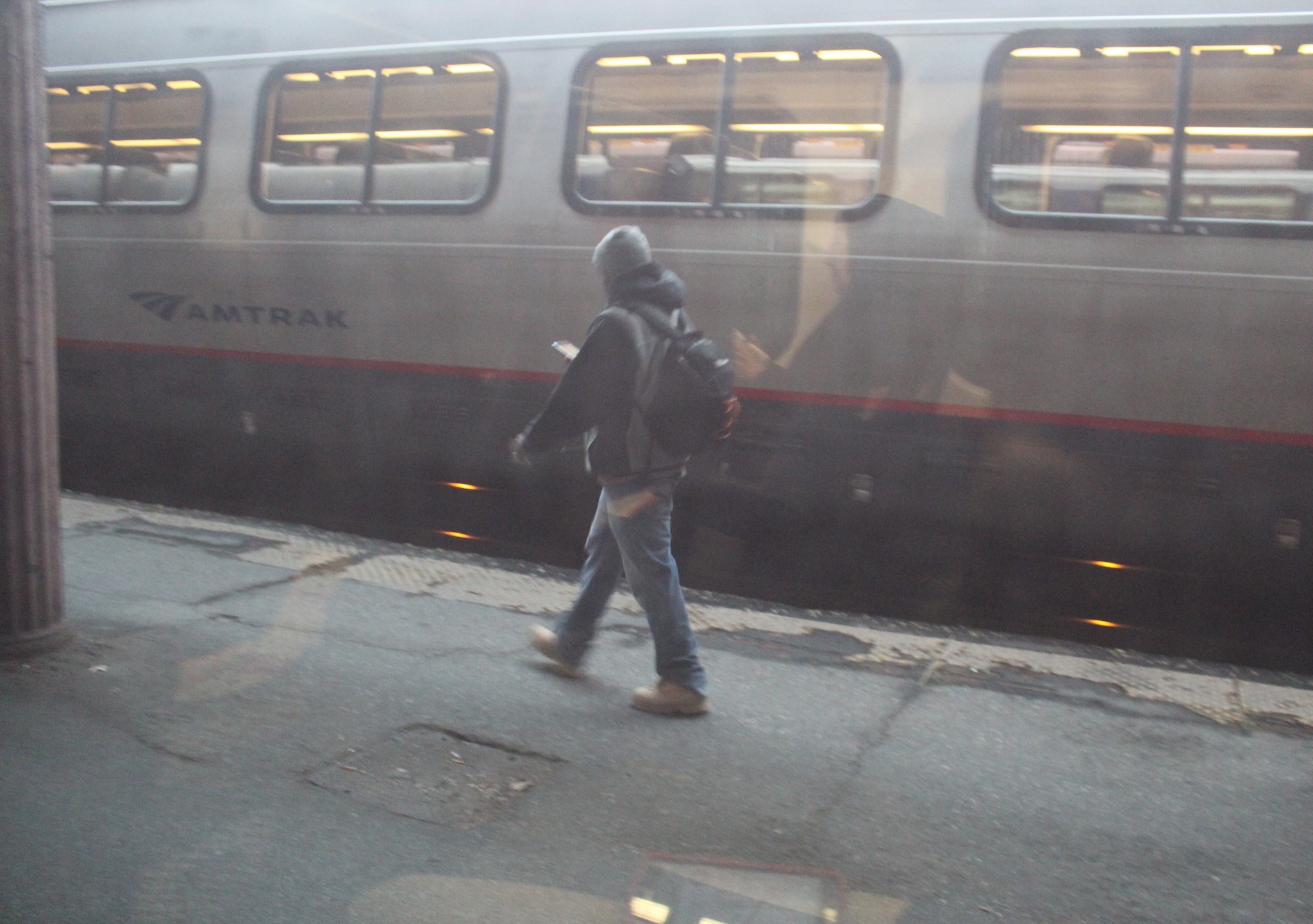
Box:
[633,675,713,713]
[528,624,586,674]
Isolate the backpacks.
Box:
[619,300,745,458]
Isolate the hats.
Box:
[592,224,653,281]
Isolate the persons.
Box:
[509,226,708,716]
[1101,133,1165,219]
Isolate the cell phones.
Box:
[552,340,580,360]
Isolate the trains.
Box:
[31,0,1313,658]
